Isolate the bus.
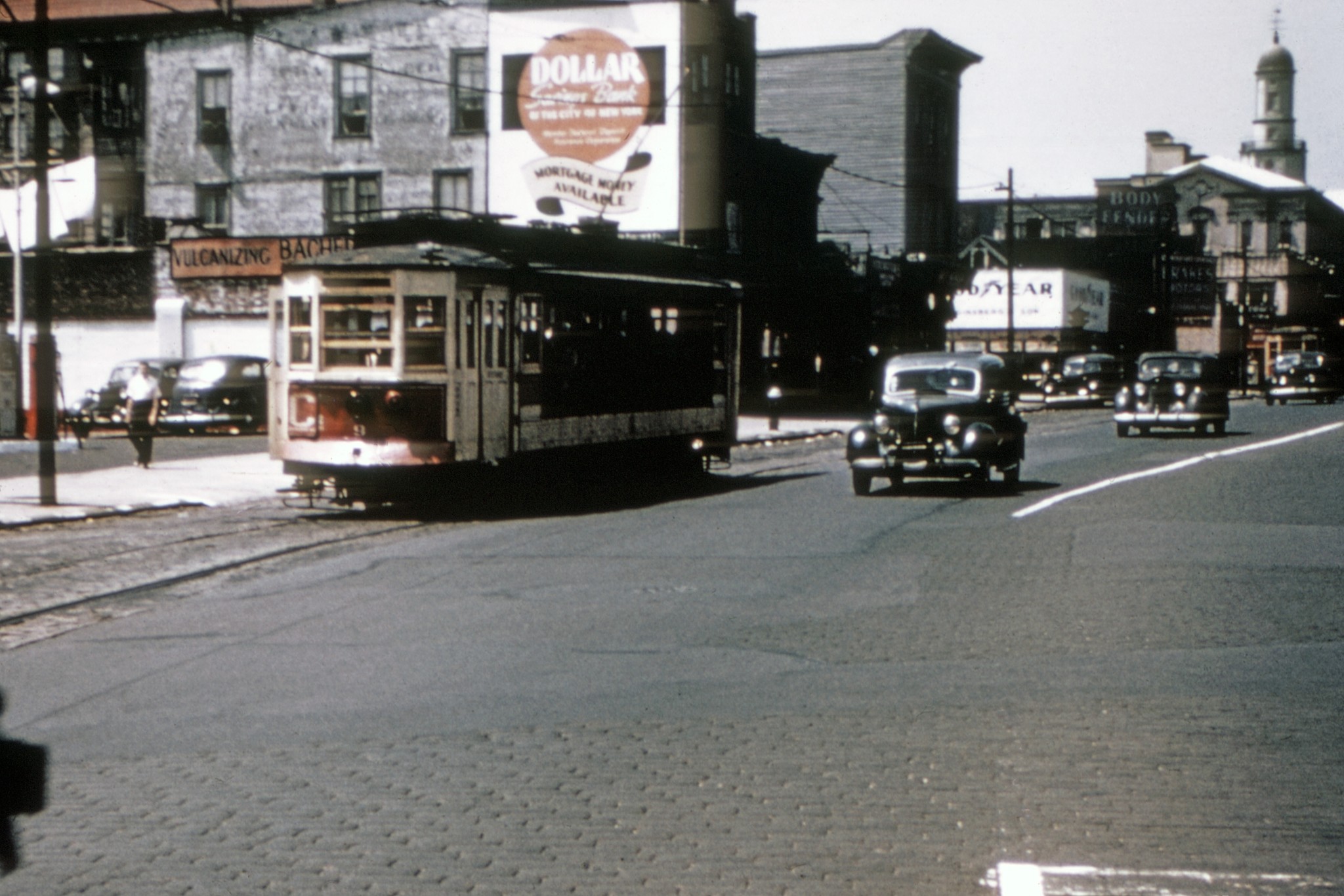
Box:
[265,205,741,515]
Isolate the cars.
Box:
[74,356,186,436]
[163,355,270,440]
[847,350,1029,497]
[1247,328,1338,405]
[1112,351,1231,438]
[1042,353,1126,398]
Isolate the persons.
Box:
[123,362,162,468]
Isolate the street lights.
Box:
[11,68,59,374]
